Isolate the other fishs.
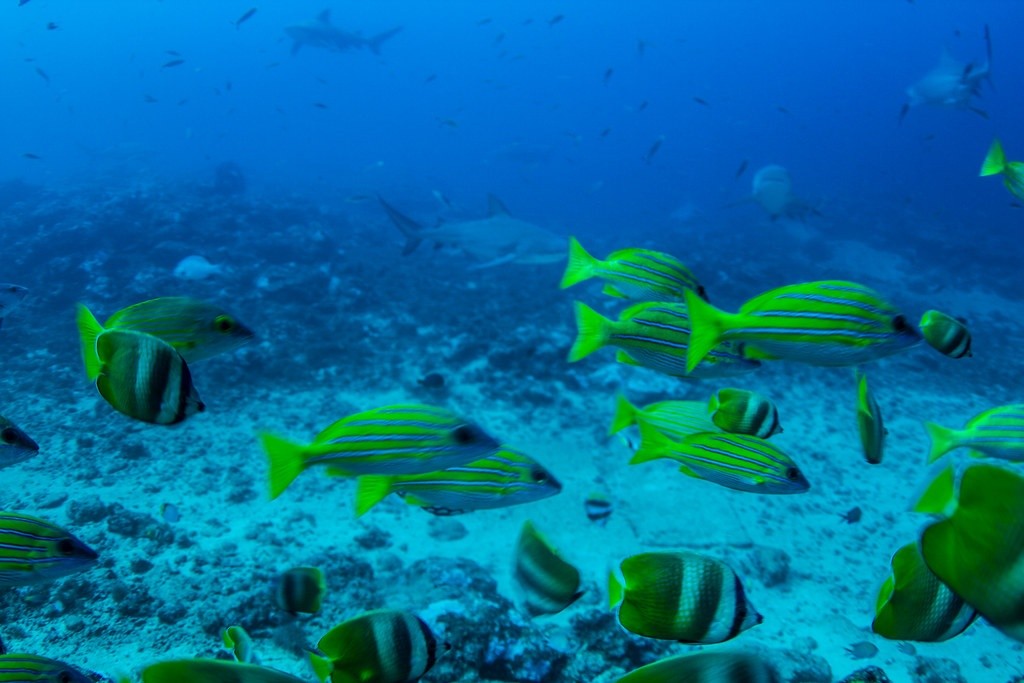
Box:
[0,0,1024,683]
